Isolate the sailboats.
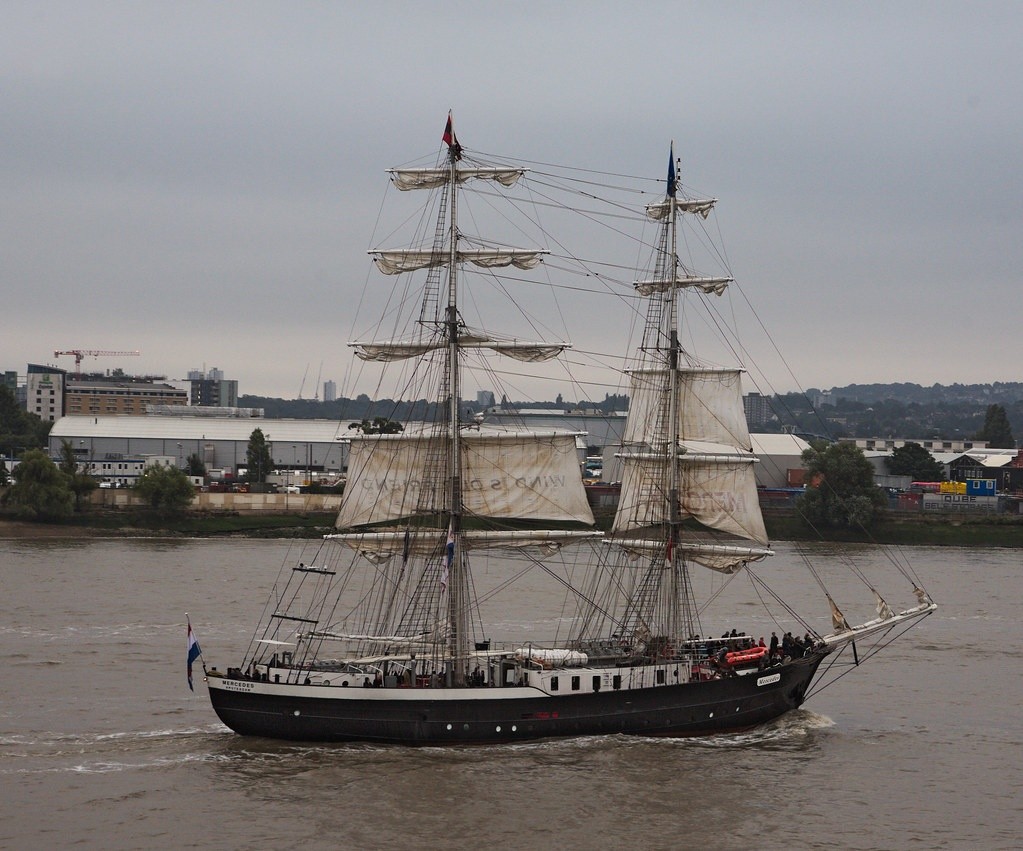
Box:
[185,110,938,747]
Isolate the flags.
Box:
[667,152,675,195]
[186,624,201,692]
[441,523,457,591]
[443,117,453,146]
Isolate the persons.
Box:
[682,629,813,667]
[269,653,280,667]
[364,666,485,688]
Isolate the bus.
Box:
[908,481,940,492]
[586,455,603,477]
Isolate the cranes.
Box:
[53,349,139,381]
[314,360,323,398]
[190,362,211,372]
[296,362,309,400]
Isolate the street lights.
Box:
[338,446,343,471]
[78,440,83,473]
[176,442,182,467]
[292,444,296,469]
[301,444,308,473]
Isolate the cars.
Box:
[583,479,621,486]
